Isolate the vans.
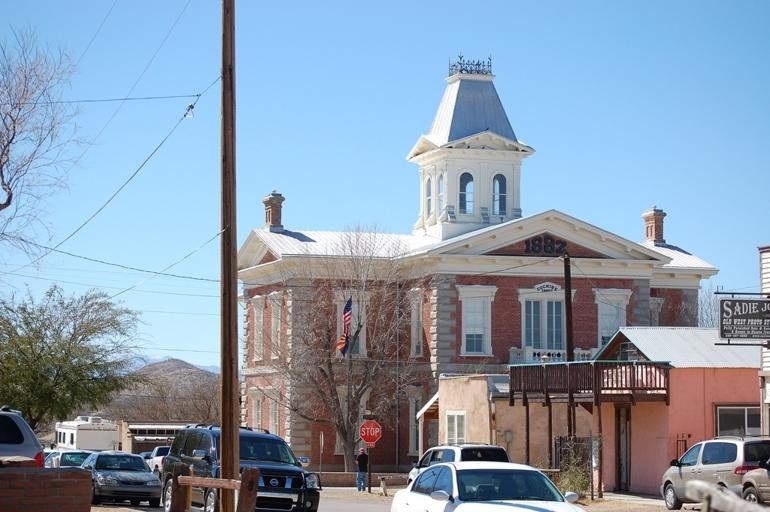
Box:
[658,434,770,510]
[408,443,511,486]
[0,404,45,467]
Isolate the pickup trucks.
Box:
[150,445,169,480]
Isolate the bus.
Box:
[128,425,190,441]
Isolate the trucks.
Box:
[50,416,120,450]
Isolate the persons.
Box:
[355,448,368,491]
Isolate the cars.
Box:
[45,450,93,467]
[140,452,152,467]
[390,460,586,512]
[740,457,770,504]
[81,450,161,507]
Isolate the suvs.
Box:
[161,424,323,511]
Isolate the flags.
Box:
[336,296,352,357]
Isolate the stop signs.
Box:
[359,420,381,443]
